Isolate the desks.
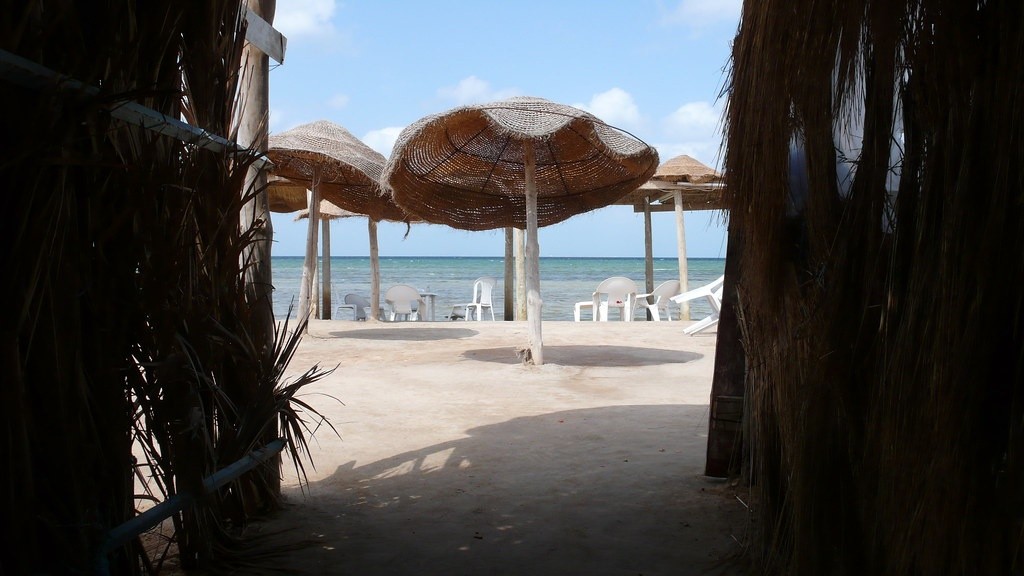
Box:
[420,293,438,322]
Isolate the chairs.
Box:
[332,283,358,321]
[632,280,680,321]
[345,294,386,321]
[592,277,637,322]
[574,300,608,322]
[448,277,497,321]
[384,284,428,321]
[669,273,725,335]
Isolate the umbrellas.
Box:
[386,97,660,366]
[266,121,418,332]
[618,154,724,320]
